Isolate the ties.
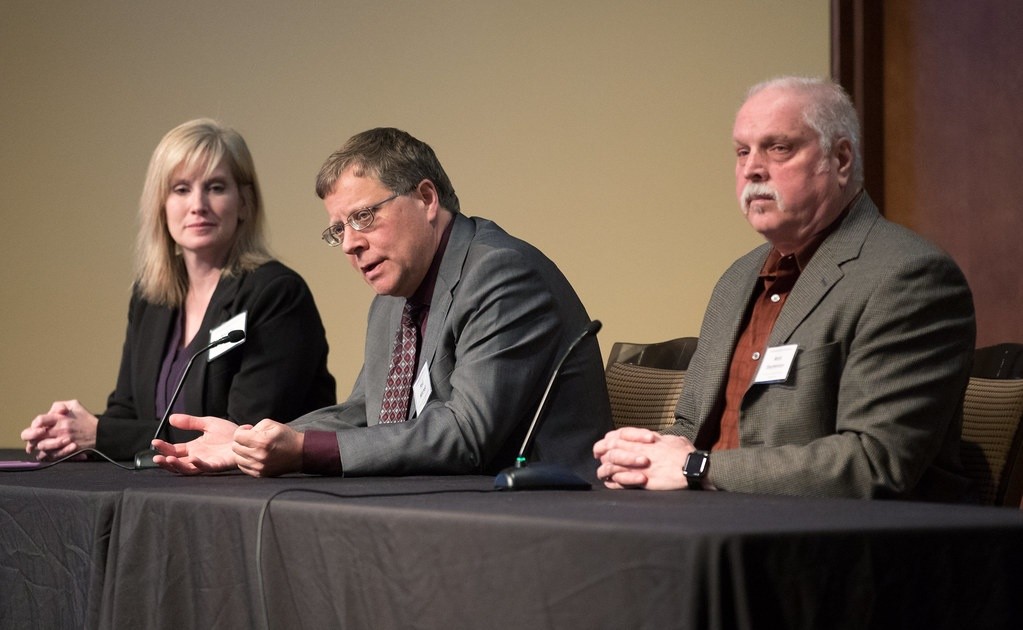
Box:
[379,288,425,426]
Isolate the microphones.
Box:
[134,329,245,469]
[494,320,602,491]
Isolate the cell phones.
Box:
[0,461,39,468]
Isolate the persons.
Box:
[21,118,336,462]
[593,78,975,498]
[152,128,615,476]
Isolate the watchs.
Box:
[682,450,710,490]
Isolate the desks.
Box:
[0,456,1023,630]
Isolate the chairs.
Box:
[604,336,699,432]
[916,342,1023,508]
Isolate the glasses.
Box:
[322,193,399,248]
